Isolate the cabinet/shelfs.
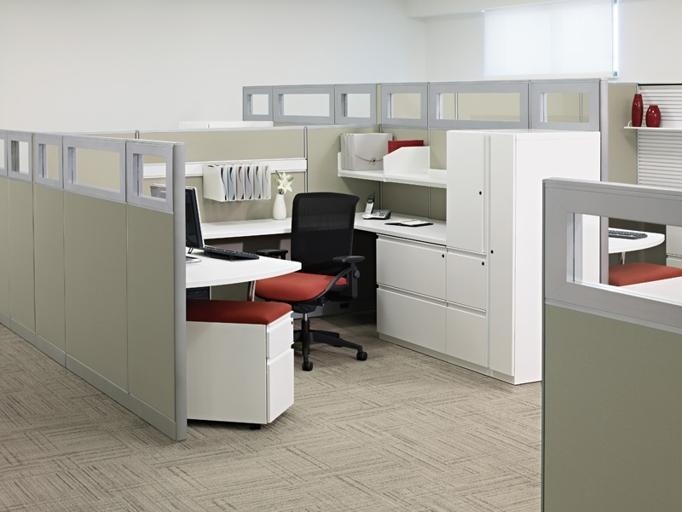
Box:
[445,306,488,369]
[446,131,486,256]
[445,248,487,314]
[375,234,447,303]
[336,123,447,240]
[375,288,446,356]
[488,131,515,376]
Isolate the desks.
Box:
[186,211,446,304]
[609,227,665,266]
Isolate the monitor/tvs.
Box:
[149,183,204,259]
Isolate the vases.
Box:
[273,195,287,220]
[646,105,661,128]
[632,94,643,127]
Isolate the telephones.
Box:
[362,193,392,219]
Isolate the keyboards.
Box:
[205,245,259,260]
[608,230,647,239]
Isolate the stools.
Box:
[610,263,682,305]
[186,300,296,428]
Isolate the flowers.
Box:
[274,170,293,195]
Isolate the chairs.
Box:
[256,192,368,372]
